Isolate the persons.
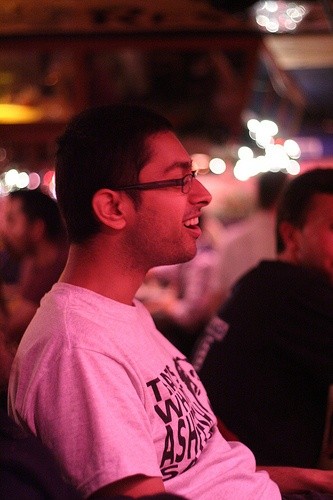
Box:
[8,104,333,500]
[193,167,333,468]
[213,172,291,301]
[0,190,69,330]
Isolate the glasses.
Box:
[106,169,199,193]
